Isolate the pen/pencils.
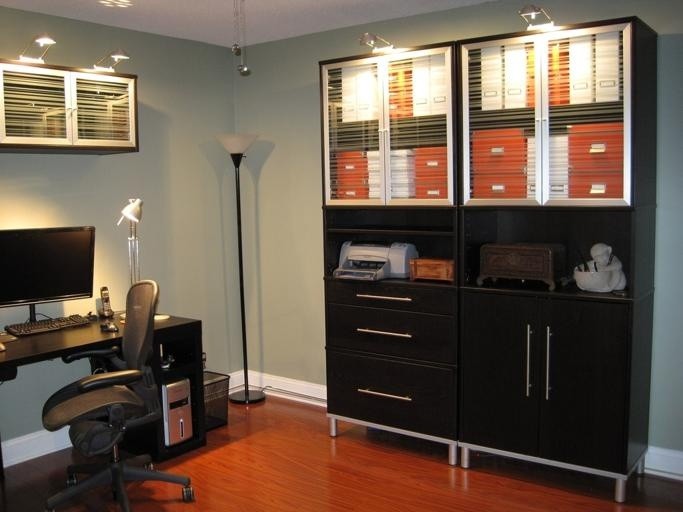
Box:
[576,249,599,272]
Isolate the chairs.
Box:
[41,279,195,512]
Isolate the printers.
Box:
[332,239,419,282]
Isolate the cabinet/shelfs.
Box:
[318,16,657,503]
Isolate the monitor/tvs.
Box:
[0,226,97,321]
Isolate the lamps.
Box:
[92,48,131,72]
[116,197,154,287]
[521,5,560,32]
[18,31,59,64]
[216,133,266,407]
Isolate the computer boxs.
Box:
[161,377,195,446]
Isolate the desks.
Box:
[0,308,208,473]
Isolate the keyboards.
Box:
[5,315,90,334]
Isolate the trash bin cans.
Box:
[203,371,230,431]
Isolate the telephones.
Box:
[99,287,114,317]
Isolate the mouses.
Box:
[100,318,118,332]
